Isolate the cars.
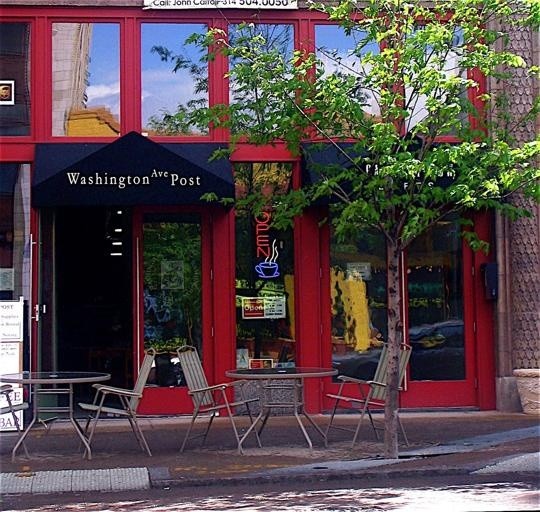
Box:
[332,320,464,380]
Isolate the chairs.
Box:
[0,385,29,459]
[326,342,413,449]
[78,348,155,459]
[176,345,262,455]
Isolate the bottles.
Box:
[240,354,245,370]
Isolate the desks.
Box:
[0,372,111,464]
[225,368,338,455]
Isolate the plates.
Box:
[259,273,280,278]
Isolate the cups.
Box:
[254,262,278,276]
[274,362,295,368]
